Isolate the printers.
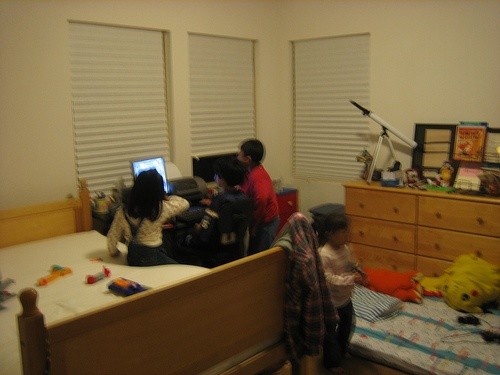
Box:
[166,176,208,207]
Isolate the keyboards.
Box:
[177,208,206,224]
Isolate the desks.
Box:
[93,188,297,259]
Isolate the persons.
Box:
[318,213,361,374]
[233,139,279,249]
[184,159,251,258]
[106,168,190,266]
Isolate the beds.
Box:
[0,179,340,375]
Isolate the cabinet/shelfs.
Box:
[343,180,500,303]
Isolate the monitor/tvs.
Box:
[131,157,169,194]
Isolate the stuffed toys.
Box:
[417,251,500,314]
[358,266,424,305]
